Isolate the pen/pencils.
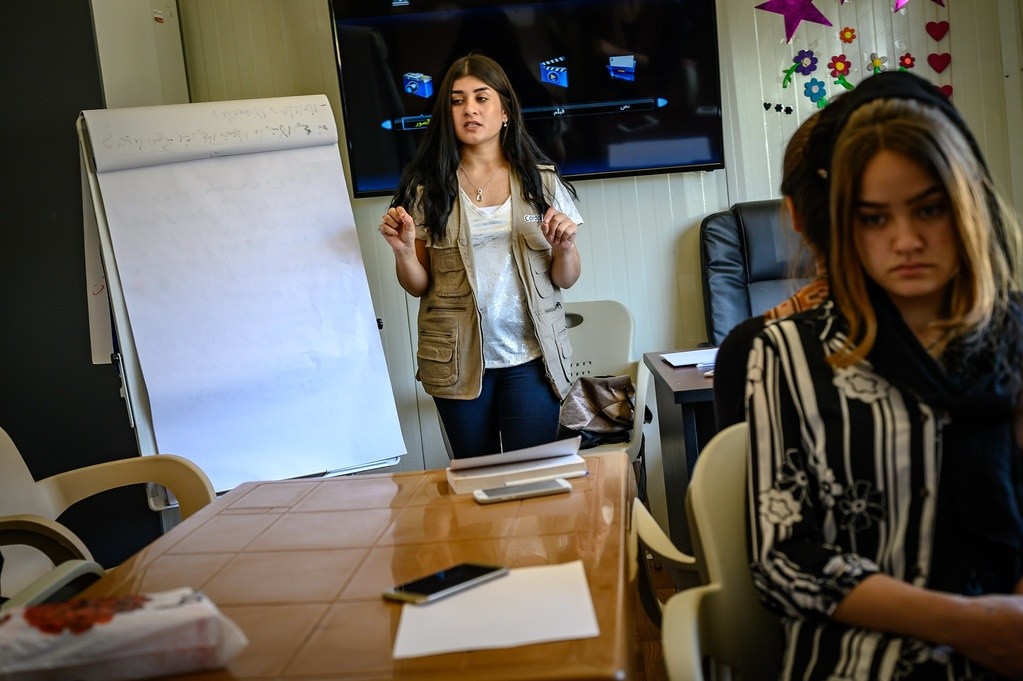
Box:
[504,471,590,488]
[704,370,713,378]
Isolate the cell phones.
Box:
[474,477,570,504]
[382,564,505,603]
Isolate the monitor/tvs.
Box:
[329,0,724,197]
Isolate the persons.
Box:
[380,55,585,461]
[709,71,1023,681]
[423,1,700,166]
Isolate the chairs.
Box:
[0,425,218,604]
[566,193,820,681]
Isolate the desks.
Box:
[644,348,716,579]
[66,450,639,681]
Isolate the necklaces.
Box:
[925,332,947,351]
[457,162,504,202]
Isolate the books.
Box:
[446,434,587,495]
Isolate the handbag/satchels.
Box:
[557,374,652,449]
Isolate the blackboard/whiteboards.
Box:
[77,96,401,511]
